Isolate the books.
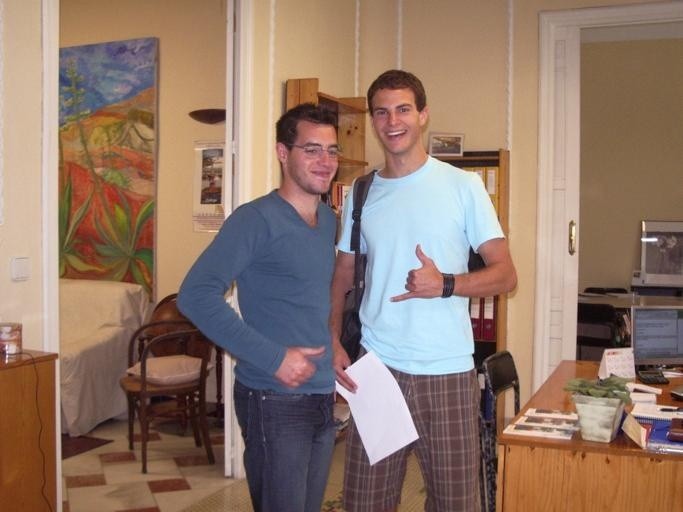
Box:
[499,382,683,453]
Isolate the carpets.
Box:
[178,440,428,512]
[61,433,114,459]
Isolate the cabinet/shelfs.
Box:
[432,149,509,364]
[286,78,367,443]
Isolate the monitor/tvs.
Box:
[631,305,683,365]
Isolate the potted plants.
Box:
[563,374,635,443]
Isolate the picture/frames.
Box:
[641,220,683,287]
[428,131,463,157]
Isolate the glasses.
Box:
[285,144,344,159]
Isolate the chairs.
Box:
[59,278,156,437]
[119,293,223,474]
[480,350,520,512]
[577,287,628,361]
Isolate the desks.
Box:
[0,349,58,512]
[495,361,683,512]
[577,294,683,311]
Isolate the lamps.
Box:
[189,109,226,124]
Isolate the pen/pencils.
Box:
[660,409,683,411]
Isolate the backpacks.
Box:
[340,286,365,371]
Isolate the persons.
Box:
[328,68,519,512]
[175,100,343,512]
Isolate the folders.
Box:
[470,296,494,339]
[462,167,498,217]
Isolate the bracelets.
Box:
[438,271,455,299]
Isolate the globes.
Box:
[145,294,221,426]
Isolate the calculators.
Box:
[638,370,669,384]
